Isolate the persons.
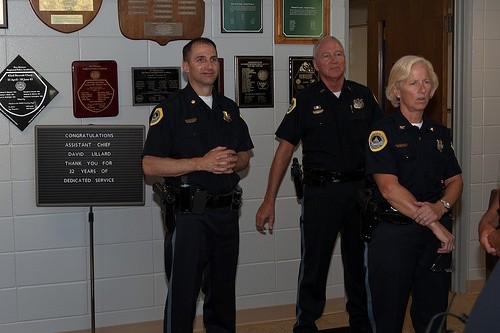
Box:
[139,35,257,318]
[465,187,500,333]
[251,35,388,333]
[361,53,465,333]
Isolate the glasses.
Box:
[430,253,455,272]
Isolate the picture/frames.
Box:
[131,66,181,106]
[289,56,320,104]
[0,0,8,29]
[220,0,263,33]
[234,55,274,108]
[273,0,330,44]
[212,58,224,95]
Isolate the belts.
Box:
[303,168,366,184]
[206,193,233,210]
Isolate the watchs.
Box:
[439,197,451,209]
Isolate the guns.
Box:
[291,158,301,175]
[151,181,173,213]
